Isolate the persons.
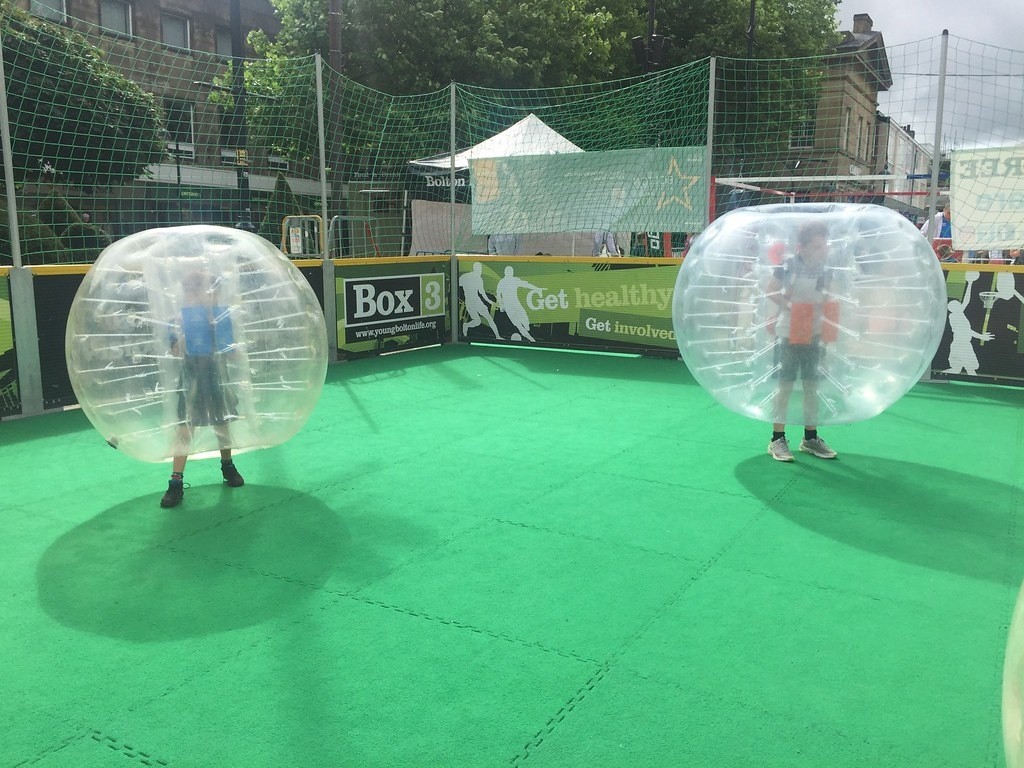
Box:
[160,269,244,507]
[919,198,952,239]
[764,220,839,461]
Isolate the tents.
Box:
[399,112,673,258]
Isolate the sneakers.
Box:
[160,480,191,507]
[799,436,837,459]
[221,464,244,487]
[767,436,795,462]
